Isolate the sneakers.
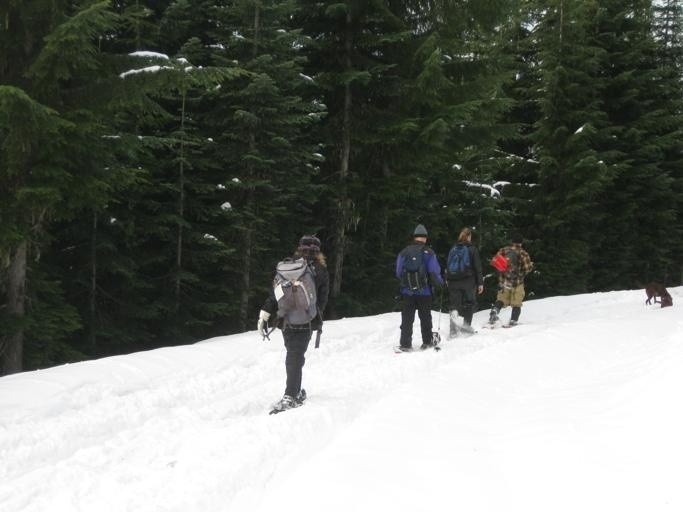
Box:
[423,307,516,346]
[281,390,305,409]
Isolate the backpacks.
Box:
[502,251,518,279]
[273,257,318,325]
[446,245,472,280]
[401,246,428,293]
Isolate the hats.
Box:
[413,224,426,237]
[511,234,522,243]
[300,235,320,247]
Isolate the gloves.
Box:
[257,310,270,341]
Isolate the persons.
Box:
[448,227,484,338]
[394,224,444,352]
[489,233,534,328]
[257,235,330,412]
